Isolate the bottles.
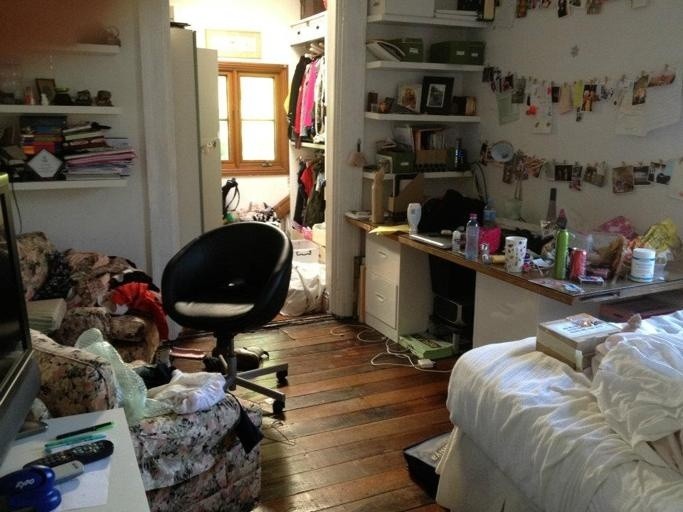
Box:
[371,173,384,224]
[630,248,656,283]
[555,226,569,280]
[465,214,479,258]
[546,188,567,230]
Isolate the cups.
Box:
[505,236,528,273]
[407,203,422,234]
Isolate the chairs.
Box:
[160,220,294,421]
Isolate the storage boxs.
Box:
[535,312,624,373]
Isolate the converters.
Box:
[418,357,434,369]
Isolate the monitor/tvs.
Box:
[0,172,43,468]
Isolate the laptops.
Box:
[408,232,465,249]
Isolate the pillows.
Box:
[16,306,146,417]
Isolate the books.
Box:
[432,0,496,21]
[17,121,135,181]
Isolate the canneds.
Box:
[568,247,587,283]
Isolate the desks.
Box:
[1,407,151,512]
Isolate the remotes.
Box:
[52,460,85,486]
[22,440,114,470]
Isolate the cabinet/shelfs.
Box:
[170,25,224,252]
[0,39,132,192]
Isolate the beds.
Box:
[447,309,683,511]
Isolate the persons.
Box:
[402,88,417,109]
[556,167,675,194]
[582,89,596,111]
[519,1,570,19]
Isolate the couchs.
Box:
[1,229,263,512]
